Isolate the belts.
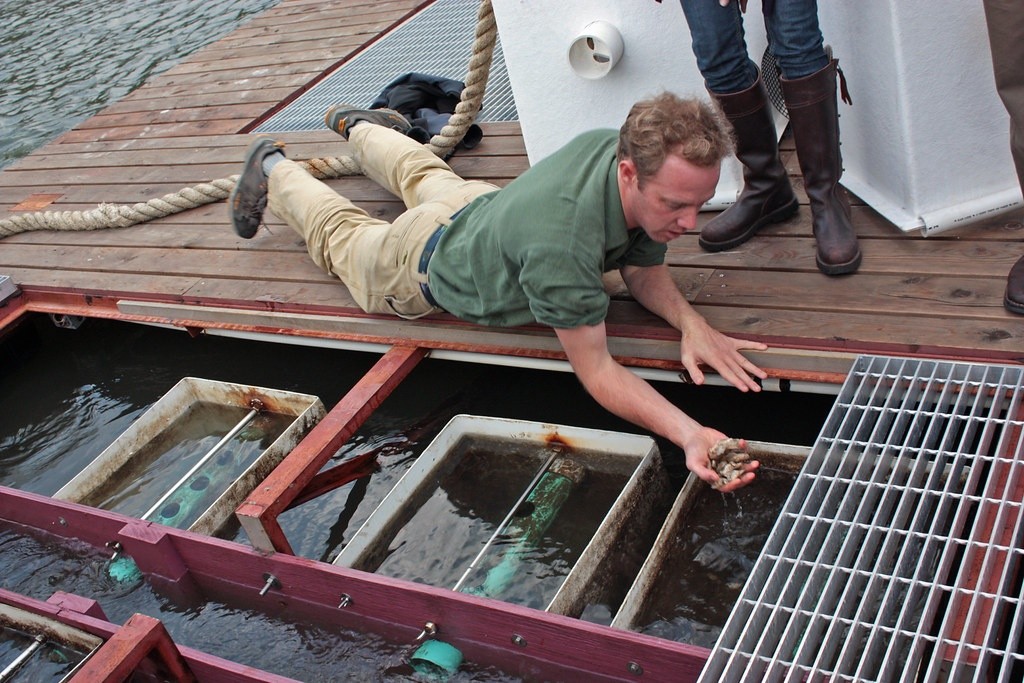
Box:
[418,203,462,314]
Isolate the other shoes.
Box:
[1004,254,1024,314]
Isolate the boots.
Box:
[698,60,799,252]
[779,58,863,276]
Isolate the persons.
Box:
[655,0,864,277]
[983,0,1024,315]
[228,91,768,491]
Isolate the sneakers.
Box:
[228,133,285,239]
[325,104,413,142]
